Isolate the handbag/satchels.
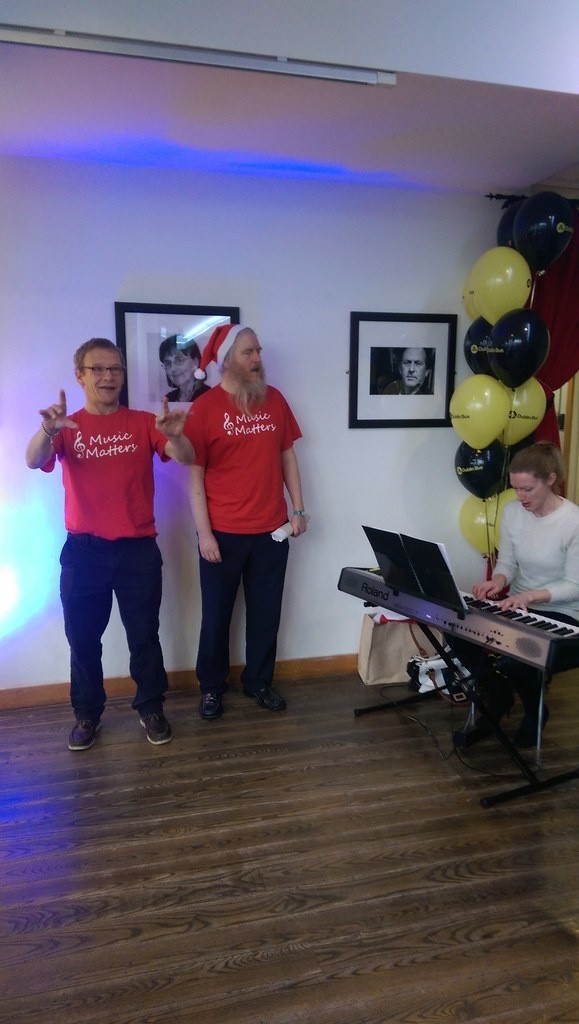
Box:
[356,612,443,686]
[406,623,471,706]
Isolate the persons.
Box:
[182,323,308,719]
[159,335,212,402]
[382,347,434,395]
[443,440,579,747]
[25,338,195,750]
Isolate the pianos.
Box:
[336,524,579,809]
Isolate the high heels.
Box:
[515,696,549,746]
[452,692,512,745]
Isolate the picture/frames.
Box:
[348,309,460,428]
[113,301,242,417]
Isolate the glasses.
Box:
[78,366,124,375]
[159,356,192,369]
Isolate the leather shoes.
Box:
[243,683,286,712]
[201,692,225,719]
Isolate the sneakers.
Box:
[140,711,172,745]
[67,717,102,750]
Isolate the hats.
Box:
[194,324,249,380]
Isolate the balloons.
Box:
[486,488,518,551]
[459,493,495,555]
[454,437,506,499]
[449,374,511,450]
[463,316,499,381]
[497,377,546,446]
[462,246,531,325]
[513,190,574,270]
[498,198,525,249]
[487,307,550,389]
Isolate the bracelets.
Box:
[293,510,304,516]
[41,418,60,444]
